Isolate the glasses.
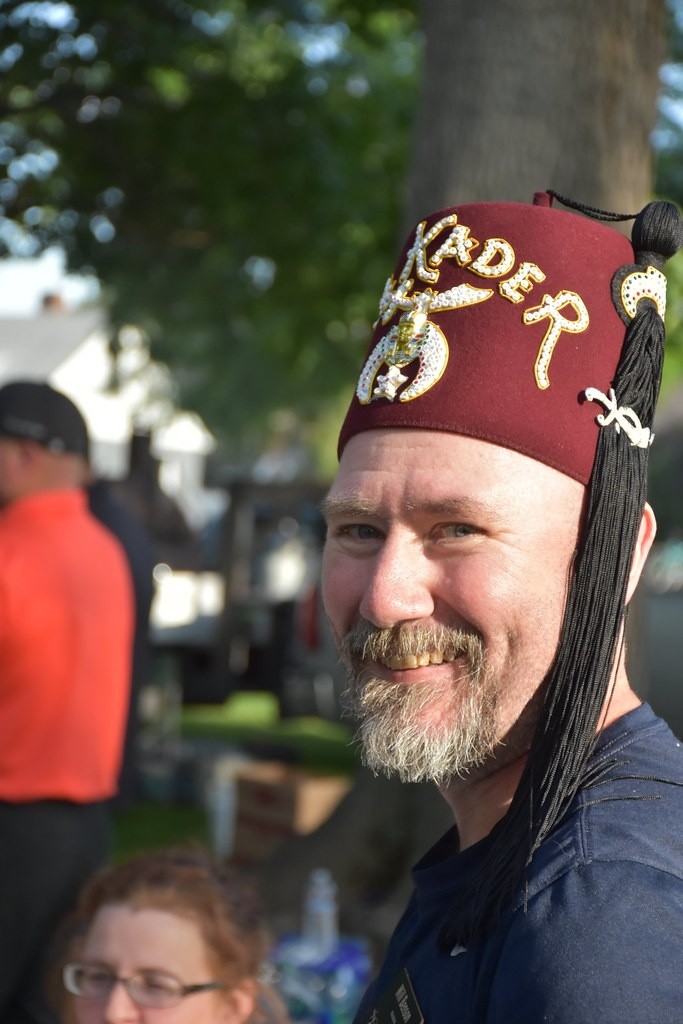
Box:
[62,960,225,1009]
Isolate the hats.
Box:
[336,201,642,496]
[0,381,89,459]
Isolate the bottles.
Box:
[301,865,337,953]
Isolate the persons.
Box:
[0,380,132,1003]
[41,844,291,1024]
[318,187,680,1021]
